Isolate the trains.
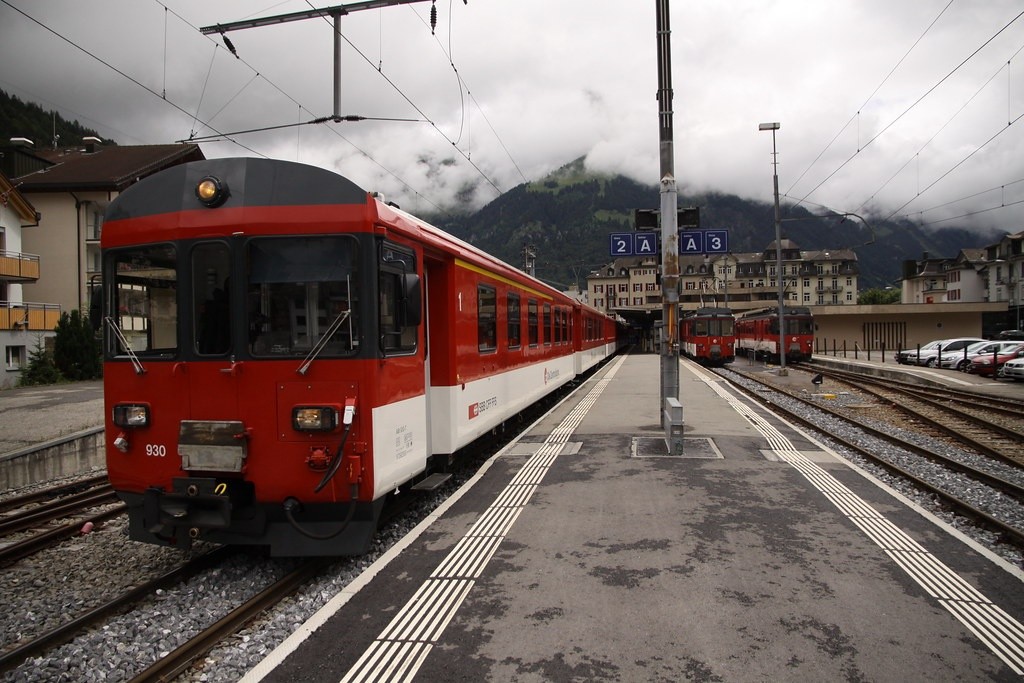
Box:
[735,306,819,363]
[679,307,736,362]
[90,157,636,558]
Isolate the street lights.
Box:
[757,121,788,376]
[995,259,1020,329]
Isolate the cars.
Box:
[934,340,1024,372]
[971,343,1024,378]
[1003,357,1024,383]
[894,336,988,368]
